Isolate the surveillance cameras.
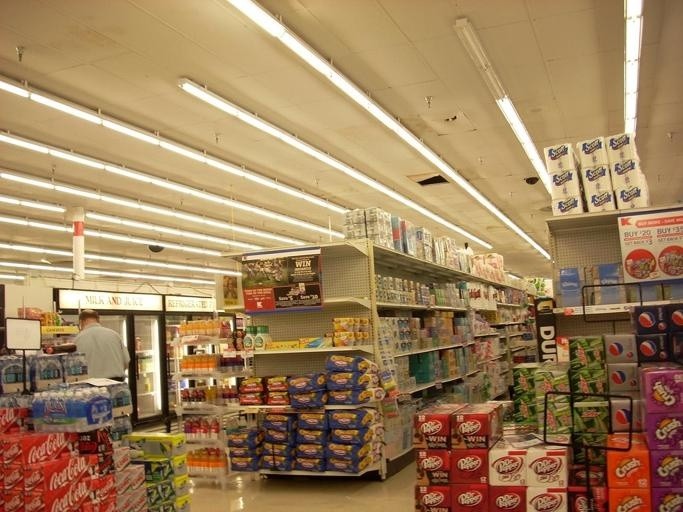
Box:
[149,245,164,252]
[526,177,540,185]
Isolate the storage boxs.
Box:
[413,332,683,512]
[0,408,189,512]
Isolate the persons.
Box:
[73,310,131,382]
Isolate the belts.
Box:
[109,377,125,381]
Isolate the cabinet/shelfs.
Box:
[544,204,683,337]
[172,236,541,482]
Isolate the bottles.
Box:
[2,351,138,439]
[177,318,270,475]
